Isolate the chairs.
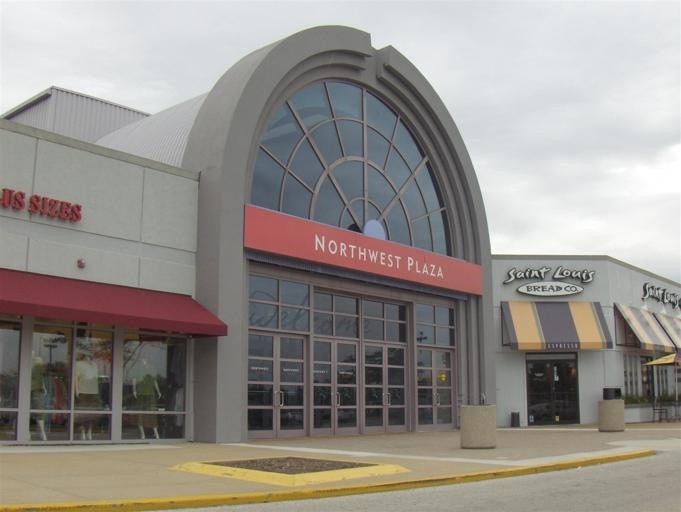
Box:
[652,401,670,423]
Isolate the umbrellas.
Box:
[641,352,681,422]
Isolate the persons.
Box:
[72,353,100,441]
[131,356,163,439]
[30,349,50,443]
